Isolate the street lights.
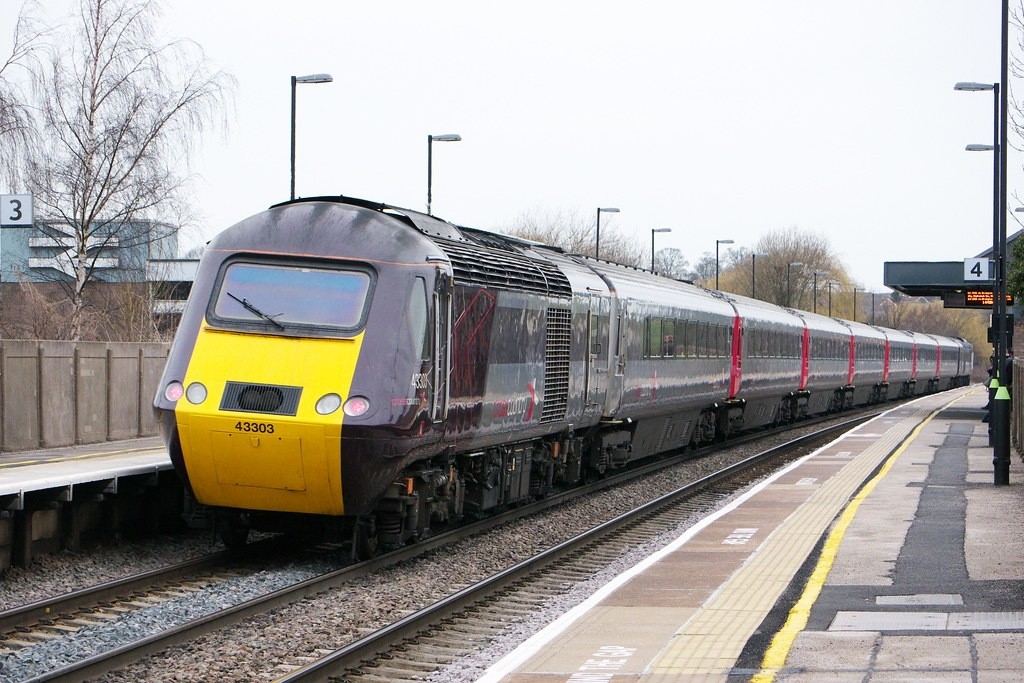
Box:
[651,227,672,271]
[426,133,462,215]
[716,239,735,290]
[813,271,828,312]
[597,205,620,261]
[827,281,841,318]
[963,142,1000,422]
[954,82,1003,446]
[788,261,803,307]
[290,74,333,199]
[752,252,770,298]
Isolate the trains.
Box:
[151,194,975,561]
